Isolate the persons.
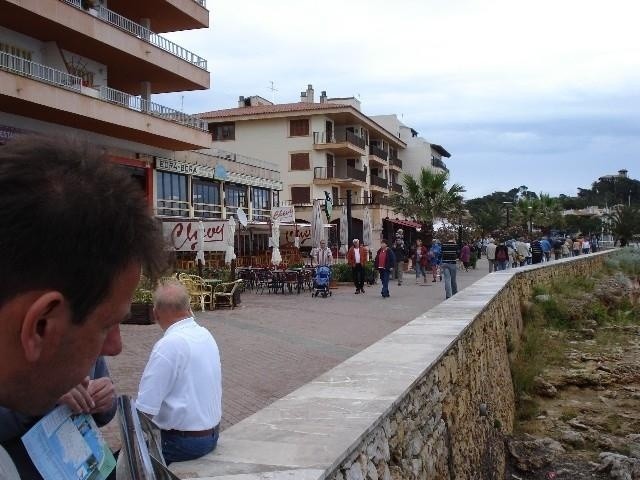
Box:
[311,239,333,266]
[57,355,117,431]
[117,285,226,463]
[0,132,168,480]
[345,226,603,299]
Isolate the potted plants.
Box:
[120,281,156,325]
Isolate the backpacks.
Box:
[497,250,506,262]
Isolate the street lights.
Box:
[502,201,514,226]
[528,206,533,232]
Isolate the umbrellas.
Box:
[224,216,236,272]
[311,198,323,248]
[196,221,205,268]
[268,218,283,269]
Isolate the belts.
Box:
[170,430,214,437]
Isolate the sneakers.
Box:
[432,280,436,282]
[361,289,365,293]
[355,291,360,294]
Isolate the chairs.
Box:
[177,247,311,312]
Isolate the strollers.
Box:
[311,265,333,299]
